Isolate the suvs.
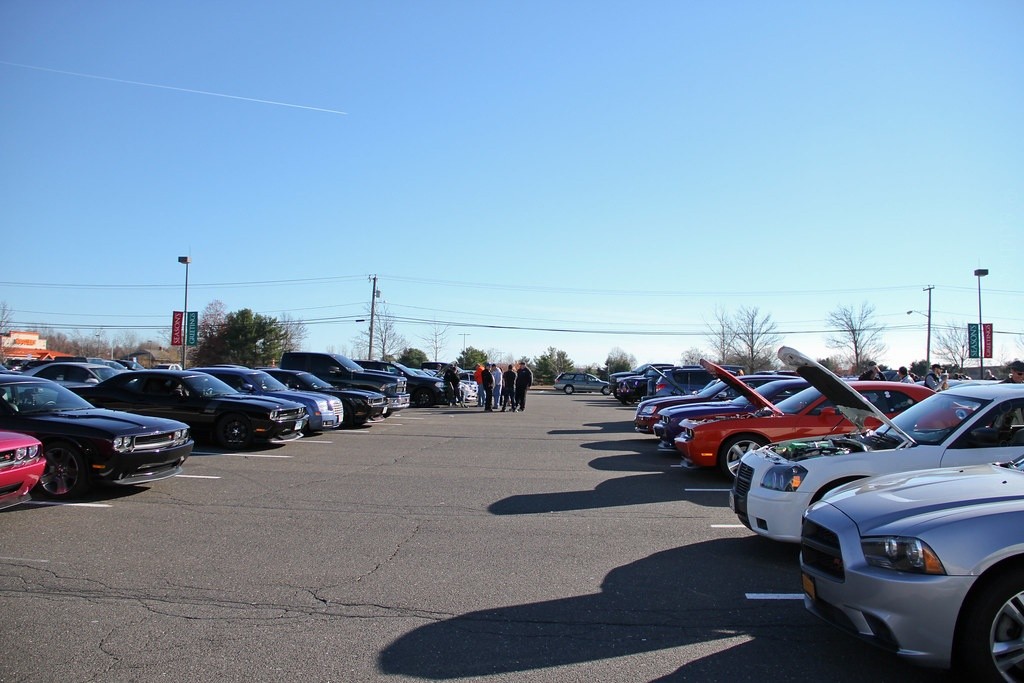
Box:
[608,364,746,406]
[421,362,456,370]
[352,360,456,408]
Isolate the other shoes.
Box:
[509,408,516,412]
[484,409,493,412]
[516,408,525,413]
[492,407,498,409]
[500,409,507,412]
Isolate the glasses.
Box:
[1012,369,1024,376]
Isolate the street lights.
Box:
[177,256,191,370]
[974,268,989,381]
[906,310,931,376]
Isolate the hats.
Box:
[931,364,945,370]
[485,363,492,366]
[519,361,525,365]
[436,364,443,367]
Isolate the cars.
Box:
[554,371,612,395]
[390,369,486,405]
[0,356,389,510]
[633,345,1024,683]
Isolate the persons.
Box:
[444,364,467,408]
[984,369,997,380]
[953,373,971,380]
[899,366,914,383]
[436,364,442,370]
[473,361,533,412]
[858,365,886,381]
[1000,361,1024,383]
[910,373,920,382]
[736,369,744,376]
[924,364,947,392]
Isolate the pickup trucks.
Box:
[252,352,411,417]
